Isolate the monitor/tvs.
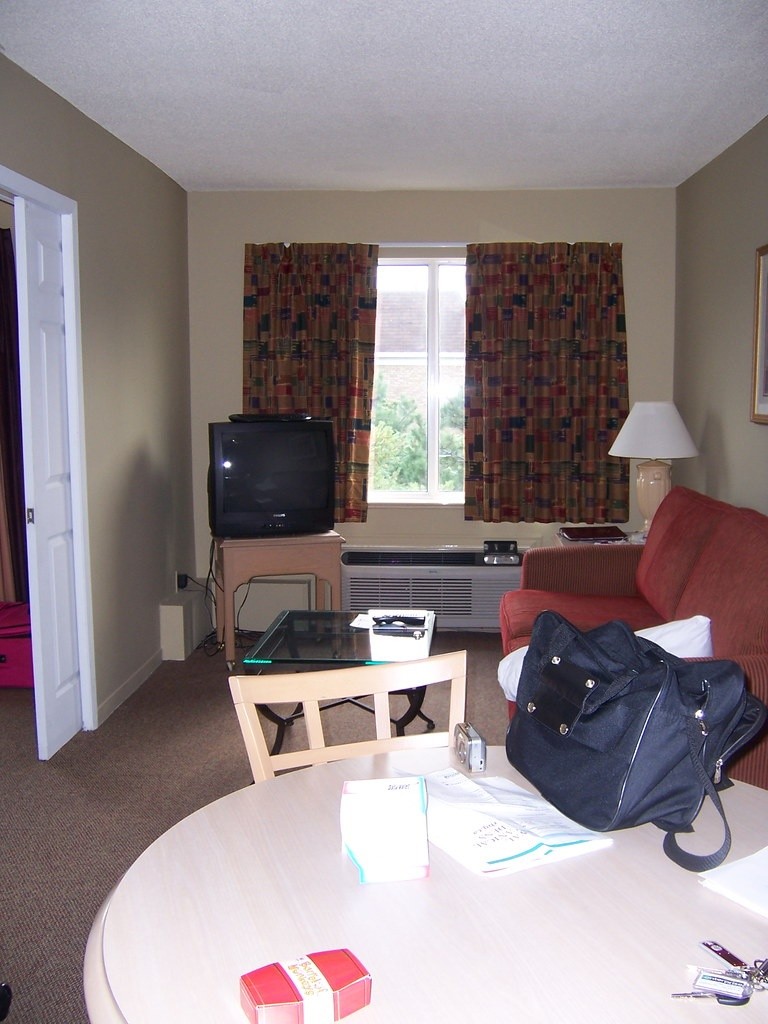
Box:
[207,418,336,539]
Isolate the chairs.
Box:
[228,650,466,783]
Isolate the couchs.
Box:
[500,486,768,790]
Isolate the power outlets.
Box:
[175,570,181,594]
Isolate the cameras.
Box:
[454,722,486,772]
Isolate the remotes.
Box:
[372,615,425,625]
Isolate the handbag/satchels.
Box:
[505,610,767,872]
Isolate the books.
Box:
[696,845,767,916]
[558,526,628,541]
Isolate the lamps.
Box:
[607,400,701,545]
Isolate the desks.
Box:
[84,747,768,1024]
[215,529,346,672]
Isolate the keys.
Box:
[670,966,753,1005]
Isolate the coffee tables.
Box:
[243,608,438,757]
